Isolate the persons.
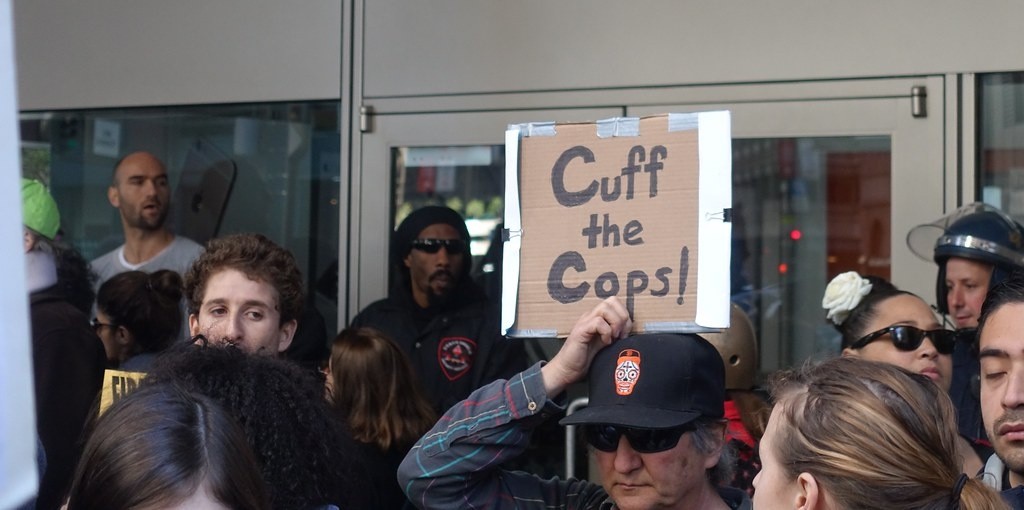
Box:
[19,148,1024,510]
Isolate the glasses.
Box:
[89,318,120,333]
[583,421,697,455]
[410,237,467,255]
[852,326,959,356]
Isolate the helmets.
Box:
[906,203,1024,317]
[696,304,757,391]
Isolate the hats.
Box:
[20,177,61,241]
[396,204,469,267]
[559,334,726,430]
